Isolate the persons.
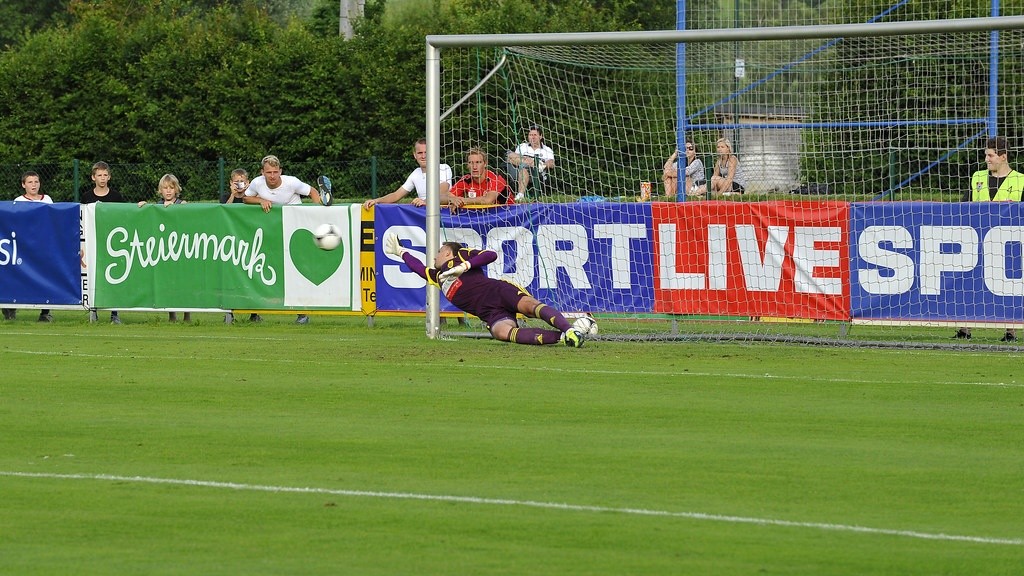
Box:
[79,162,126,326]
[693,137,745,196]
[363,137,468,326]
[243,156,334,327]
[950,136,1024,342]
[385,232,585,348]
[507,127,557,203]
[662,138,706,200]
[1,172,55,321]
[439,146,527,328]
[218,169,263,325]
[137,174,192,325]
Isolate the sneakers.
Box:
[1,308,17,320]
[111,314,121,324]
[38,313,54,324]
[295,316,308,325]
[564,329,584,349]
[316,174,332,206]
[950,331,972,341]
[249,315,262,322]
[1001,332,1018,343]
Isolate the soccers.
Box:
[313,223,343,251]
[571,317,599,336]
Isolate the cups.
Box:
[640,181,652,201]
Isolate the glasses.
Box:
[686,146,694,151]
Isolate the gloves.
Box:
[384,233,403,256]
[438,265,465,282]
